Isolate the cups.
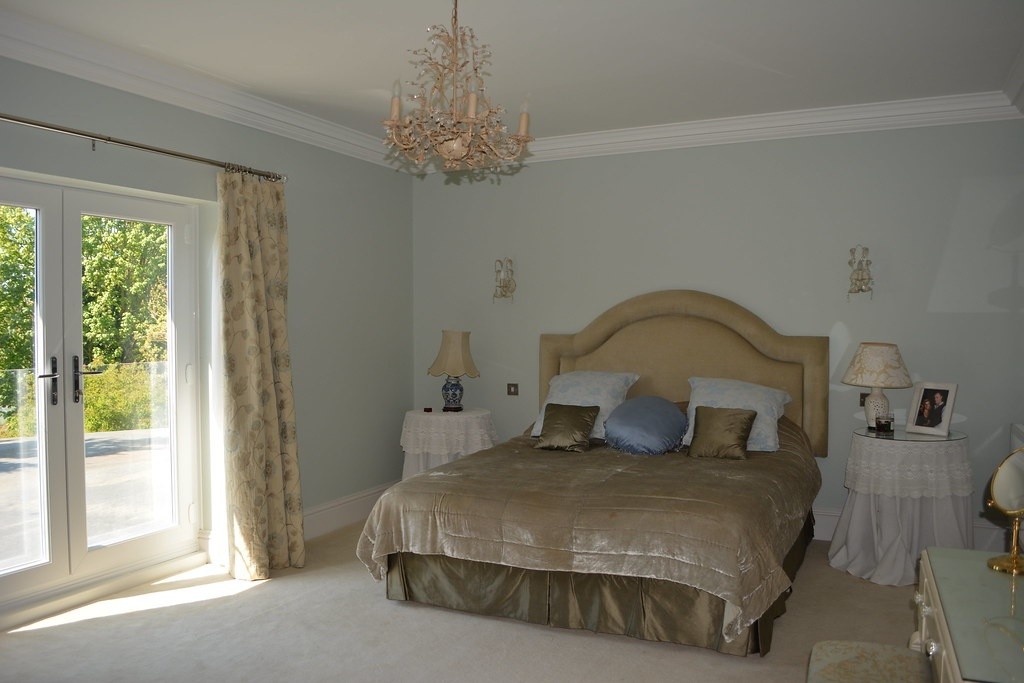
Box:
[874,411,895,440]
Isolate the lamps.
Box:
[492,257,516,304]
[427,329,481,412]
[841,342,914,433]
[378,0,536,174]
[846,243,875,301]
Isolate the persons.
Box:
[916,392,946,428]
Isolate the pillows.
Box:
[682,377,794,452]
[688,405,758,462]
[603,395,689,456]
[531,370,641,440]
[535,402,600,453]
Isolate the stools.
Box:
[971,512,1011,552]
[808,640,934,683]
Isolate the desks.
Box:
[403,409,491,481]
[852,426,970,583]
[908,546,1024,683]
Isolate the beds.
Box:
[353,288,831,658]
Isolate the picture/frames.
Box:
[905,382,959,437]
